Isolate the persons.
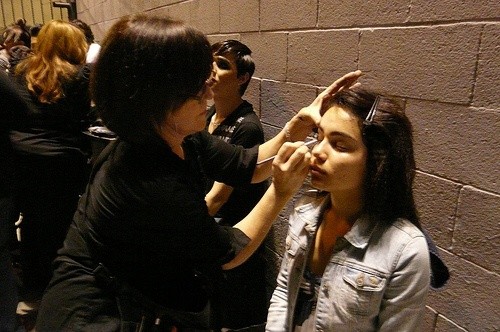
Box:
[0,17,105,78]
[0,20,101,332]
[187,40,267,332]
[34,11,366,332]
[262,85,434,332]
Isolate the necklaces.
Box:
[211,122,221,128]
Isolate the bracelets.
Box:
[284,122,296,144]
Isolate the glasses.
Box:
[180,70,215,100]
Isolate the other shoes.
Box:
[15,311,38,332]
